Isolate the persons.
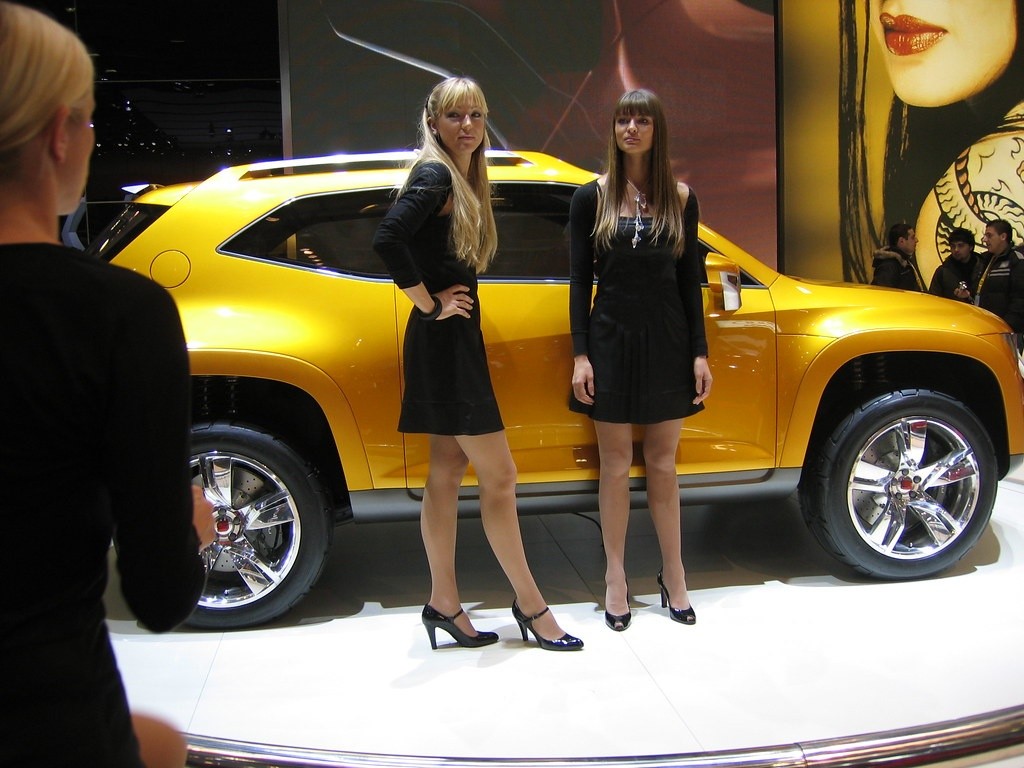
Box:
[928,228,980,305]
[871,224,928,293]
[0,4,216,768]
[839,0,1024,289]
[954,220,1024,356]
[568,90,712,629]
[372,76,584,651]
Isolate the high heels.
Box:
[422,603,499,649]
[656,566,696,624]
[605,570,631,631]
[511,597,584,650]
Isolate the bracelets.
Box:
[414,297,442,322]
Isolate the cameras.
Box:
[959,281,968,291]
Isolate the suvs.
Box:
[84,149,1020,632]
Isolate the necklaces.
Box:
[624,175,652,248]
